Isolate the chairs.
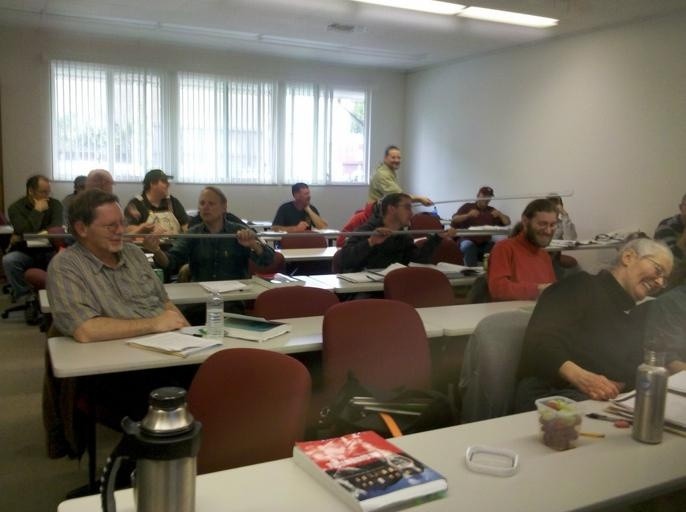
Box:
[320,299,432,407]
[414,237,464,265]
[384,267,455,307]
[411,212,447,230]
[280,234,328,248]
[469,309,534,420]
[259,229,340,241]
[254,286,339,320]
[185,348,313,477]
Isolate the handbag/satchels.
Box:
[309,370,457,443]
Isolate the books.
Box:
[544,239,578,249]
[592,239,619,245]
[198,281,256,298]
[219,313,290,343]
[606,369,686,436]
[336,271,385,283]
[251,272,306,290]
[125,331,221,358]
[408,261,483,278]
[468,225,509,231]
[291,430,448,512]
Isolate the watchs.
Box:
[250,240,261,251]
[150,247,160,252]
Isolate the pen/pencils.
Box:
[579,432,604,438]
[180,332,202,337]
[364,275,375,281]
[586,413,632,424]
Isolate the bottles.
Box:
[206,288,224,342]
[633,344,669,445]
[431,206,438,217]
[483,253,490,271]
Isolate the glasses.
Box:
[643,254,670,289]
[32,189,54,199]
[396,202,413,213]
[82,217,124,232]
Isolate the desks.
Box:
[47,309,445,483]
[545,238,617,252]
[26,238,50,248]
[39,266,487,314]
[274,246,343,262]
[56,393,686,512]
[444,299,540,337]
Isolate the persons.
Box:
[124,169,189,253]
[367,146,433,207]
[270,182,328,249]
[336,200,378,248]
[332,193,455,301]
[648,193,686,296]
[60,175,86,226]
[142,186,276,327]
[546,191,577,240]
[487,198,559,303]
[46,187,191,497]
[85,169,113,193]
[514,237,675,412]
[635,282,686,371]
[187,209,274,252]
[450,187,511,268]
[2,175,66,324]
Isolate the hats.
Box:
[143,169,176,184]
[479,186,496,196]
